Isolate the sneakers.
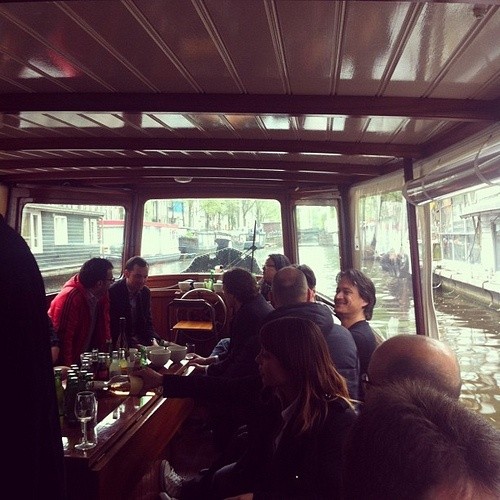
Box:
[159,459,185,500]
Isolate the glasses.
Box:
[262,264,277,269]
[100,277,116,284]
[361,374,381,392]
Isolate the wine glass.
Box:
[74,391,97,451]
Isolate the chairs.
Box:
[168,298,216,352]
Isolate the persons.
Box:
[334,269,376,377]
[364,333,463,411]
[341,378,500,500]
[0,214,72,500]
[47,258,115,367]
[129,317,353,500]
[186,269,273,435]
[108,256,162,351]
[226,267,361,400]
[256,254,316,305]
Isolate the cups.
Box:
[186,343,195,360]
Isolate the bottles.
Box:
[211,271,215,282]
[119,348,128,376]
[54,348,109,418]
[109,351,122,379]
[86,375,143,396]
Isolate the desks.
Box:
[61,359,195,500]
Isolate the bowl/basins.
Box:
[193,282,205,288]
[149,349,171,367]
[213,284,223,291]
[179,282,191,289]
[169,346,187,363]
